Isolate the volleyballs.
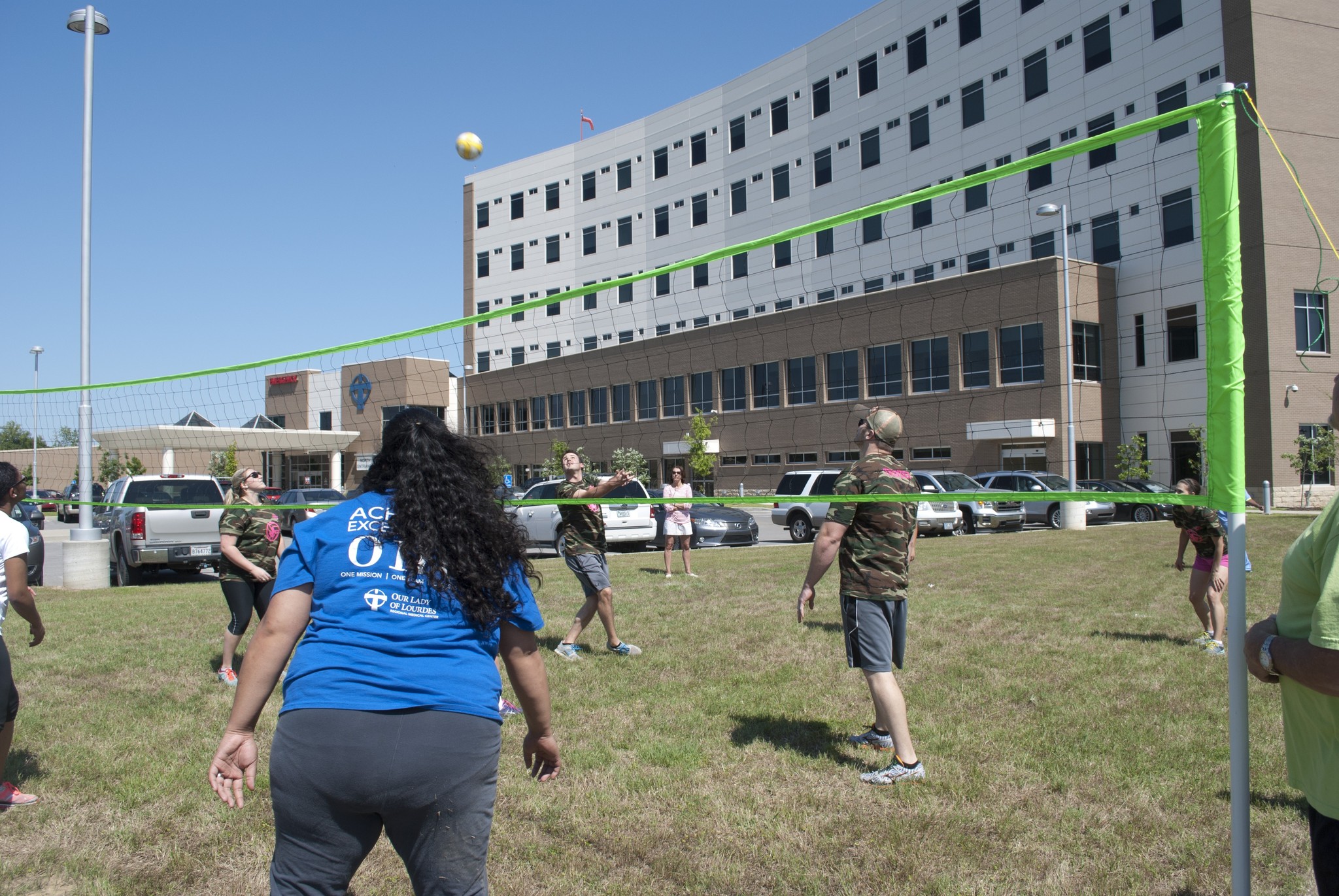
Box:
[455,132,484,160]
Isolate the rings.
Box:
[217,773,225,778]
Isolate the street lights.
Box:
[28,345,45,512]
[462,365,474,437]
[1035,202,1085,530]
[67,5,104,541]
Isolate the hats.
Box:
[852,403,902,445]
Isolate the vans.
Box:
[771,467,964,545]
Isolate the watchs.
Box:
[1259,634,1282,676]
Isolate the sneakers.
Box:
[278,670,287,681]
[217,665,238,687]
[1198,641,1225,656]
[1193,630,1214,646]
[554,640,584,662]
[498,699,523,719]
[606,639,642,656]
[848,722,896,752]
[859,754,927,789]
[0,781,39,810]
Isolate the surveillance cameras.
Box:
[1291,385,1299,393]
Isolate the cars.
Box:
[1053,477,1178,523]
[646,488,760,551]
[9,489,64,587]
[260,486,349,537]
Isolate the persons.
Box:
[208,407,562,896]
[797,403,927,789]
[553,449,645,661]
[492,651,524,715]
[216,467,287,685]
[0,462,46,807]
[1244,374,1339,896]
[662,465,698,579]
[1171,478,1264,656]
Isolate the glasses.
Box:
[858,418,879,440]
[9,474,28,497]
[672,471,681,475]
[242,472,262,483]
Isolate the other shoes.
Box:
[685,572,698,578]
[665,574,671,578]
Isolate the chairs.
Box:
[181,489,193,500]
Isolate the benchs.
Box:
[306,497,339,501]
[136,496,210,504]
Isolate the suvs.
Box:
[56,482,106,523]
[969,470,1117,530]
[907,469,1026,535]
[91,473,236,587]
[490,472,657,557]
